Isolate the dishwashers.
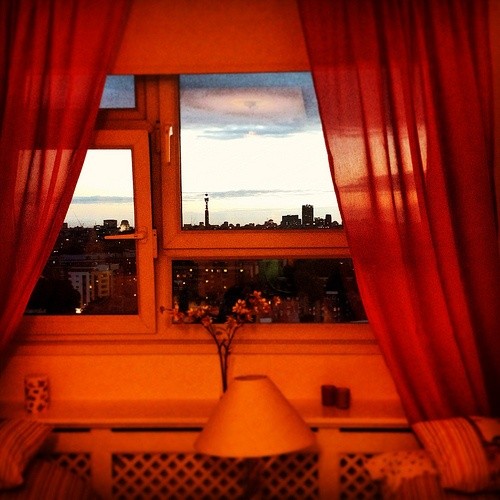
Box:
[335,386,350,409]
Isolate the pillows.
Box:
[410,415,499,494]
[366,448,451,499]
[1,457,104,499]
[0,417,55,489]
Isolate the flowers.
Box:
[160,292,284,391]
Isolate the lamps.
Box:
[193,375,316,500]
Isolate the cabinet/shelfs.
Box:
[1,400,443,499]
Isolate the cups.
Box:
[321,384,337,406]
[24,375,49,414]
